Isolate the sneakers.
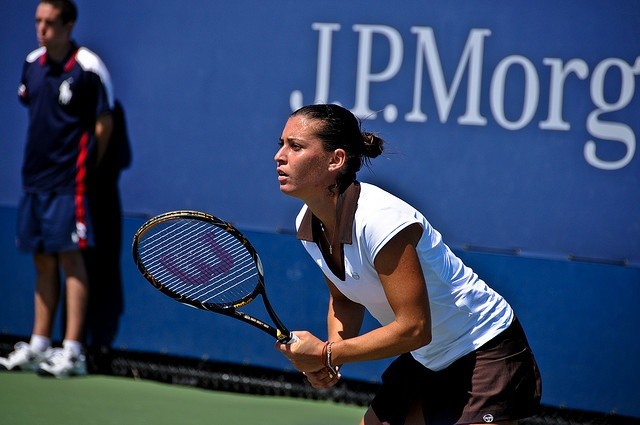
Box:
[36,347,88,378]
[0,341,54,372]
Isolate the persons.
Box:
[273,104,542,424]
[0,0,114,376]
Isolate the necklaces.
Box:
[318,222,338,254]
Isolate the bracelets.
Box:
[322,340,329,378]
[326,342,343,374]
